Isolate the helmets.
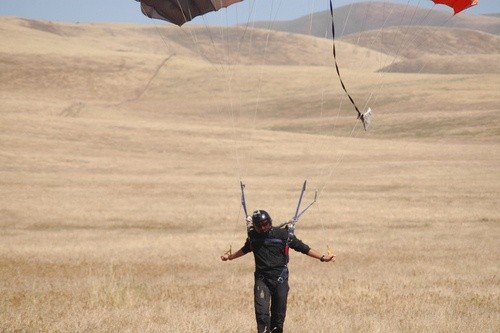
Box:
[251,210,269,224]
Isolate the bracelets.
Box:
[320,255,325,262]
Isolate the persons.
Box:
[220,210,335,333]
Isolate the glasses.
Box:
[253,220,269,229]
